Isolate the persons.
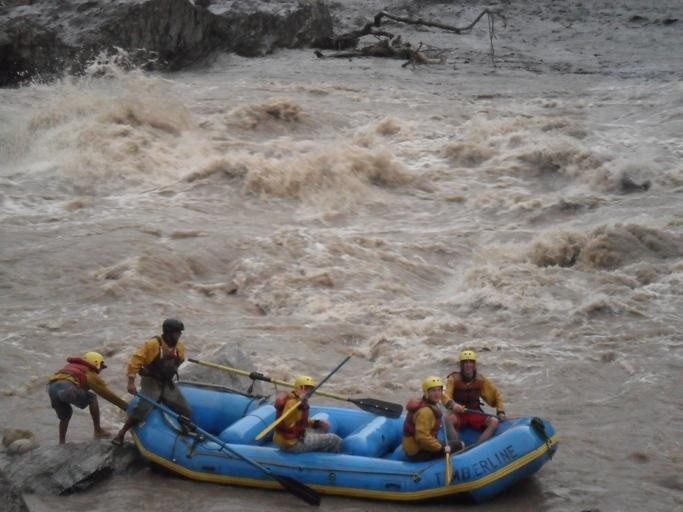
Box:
[46,319,193,445]
[403,350,506,461]
[272,376,343,452]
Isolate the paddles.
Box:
[255,353,353,442]
[188,357,403,419]
[439,401,454,487]
[136,391,321,505]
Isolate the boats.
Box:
[126,381,561,503]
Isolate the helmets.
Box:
[459,351,478,362]
[83,352,104,370]
[423,377,444,391]
[163,319,184,331]
[295,376,315,389]
